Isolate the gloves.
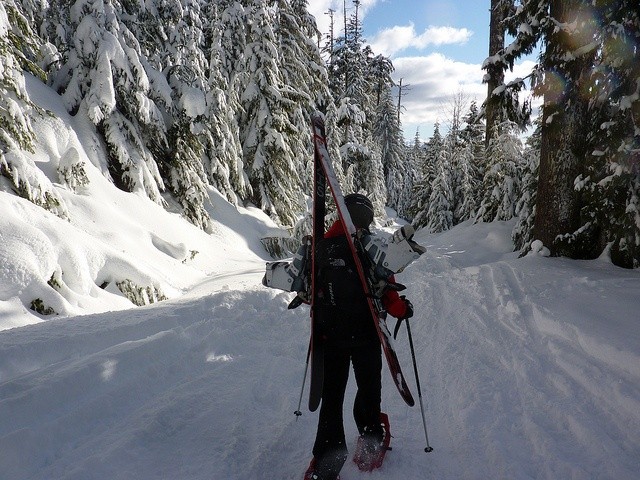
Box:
[401,294,414,319]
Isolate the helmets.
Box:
[344,193,375,230]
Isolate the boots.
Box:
[262,243,308,292]
[361,224,426,279]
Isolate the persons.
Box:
[295,193,413,480]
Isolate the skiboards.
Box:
[305,412,392,480]
[308,113,416,411]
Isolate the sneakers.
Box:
[364,423,386,453]
